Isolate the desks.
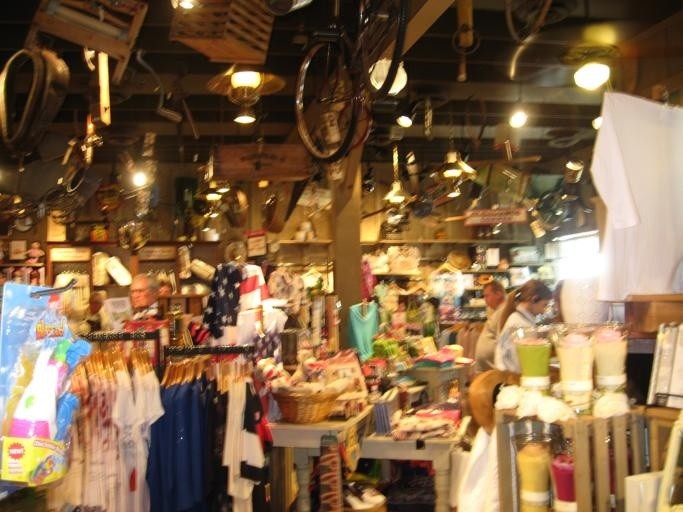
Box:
[267,401,375,511]
[361,396,474,512]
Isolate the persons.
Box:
[84,292,105,331]
[393,301,408,322]
[130,272,160,320]
[473,281,506,373]
[495,280,552,374]
[160,281,174,295]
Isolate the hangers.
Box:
[447,321,485,332]
[160,344,254,395]
[73,328,155,381]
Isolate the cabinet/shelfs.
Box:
[42,239,553,331]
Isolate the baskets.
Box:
[273,391,337,424]
